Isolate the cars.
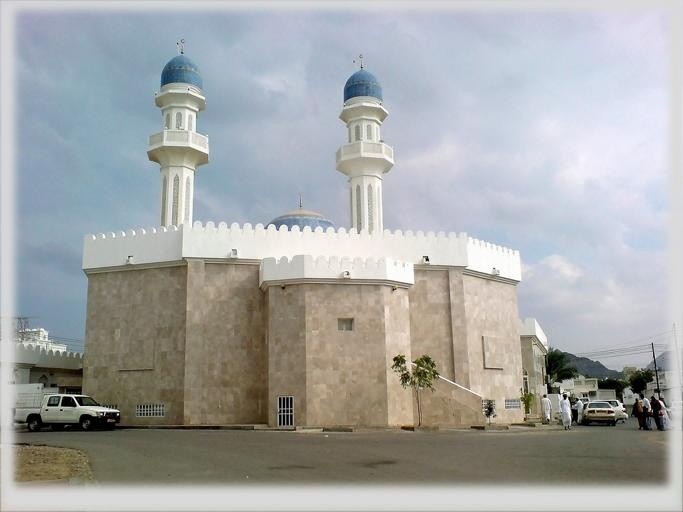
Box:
[571,398,629,426]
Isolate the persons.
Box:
[572,397,584,424]
[560,393,573,430]
[540,394,552,423]
[631,393,670,431]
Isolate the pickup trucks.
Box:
[13,392,120,430]
